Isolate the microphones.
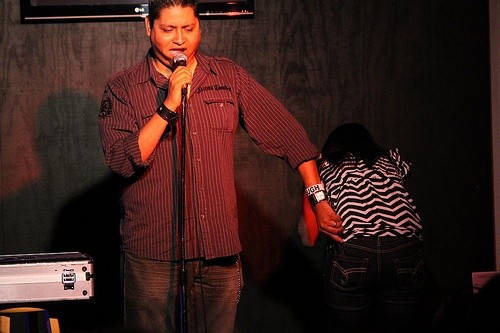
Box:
[174,53,188,96]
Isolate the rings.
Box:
[334,220,337,227]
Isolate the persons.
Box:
[297,122,423,333]
[99,0,344,333]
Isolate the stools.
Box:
[0,303,60,333]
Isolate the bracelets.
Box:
[156,103,179,125]
[305,184,327,206]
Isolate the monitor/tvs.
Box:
[18,0,254,25]
